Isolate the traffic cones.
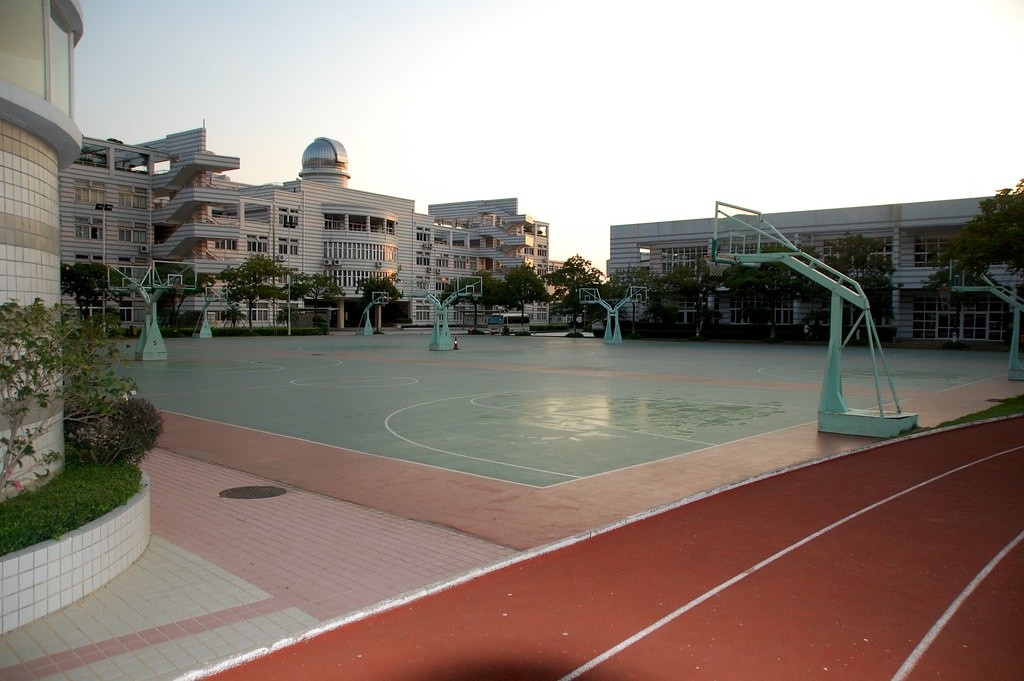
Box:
[453,335,462,350]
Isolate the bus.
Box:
[487,312,530,334]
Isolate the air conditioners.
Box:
[429,243,433,248]
[140,245,149,253]
[278,254,286,261]
[323,259,332,265]
[423,243,429,248]
[428,267,432,272]
[334,259,342,265]
[436,268,442,273]
[377,260,383,267]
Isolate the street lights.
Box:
[95,203,114,339]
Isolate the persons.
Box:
[696,317,777,344]
[804,321,814,340]
